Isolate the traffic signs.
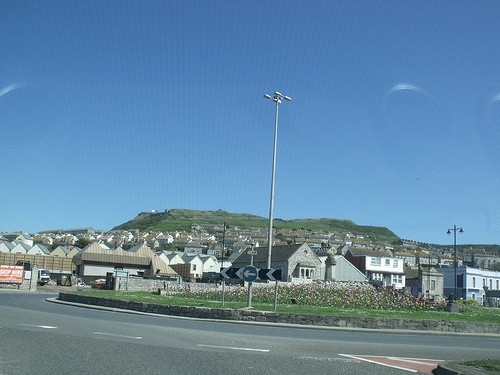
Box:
[220,268,283,282]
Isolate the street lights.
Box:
[446,225,464,299]
[262,92,292,268]
[221,223,229,268]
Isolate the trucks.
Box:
[37,268,51,286]
